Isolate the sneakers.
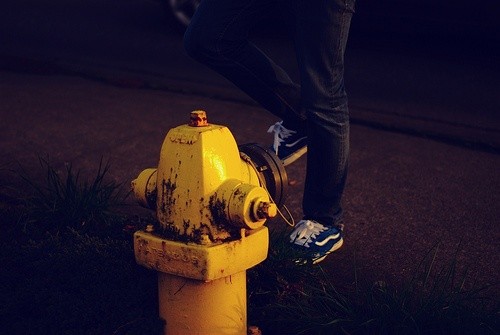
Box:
[287,219,344,267]
[267,120,308,167]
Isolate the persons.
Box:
[182,0,355,266]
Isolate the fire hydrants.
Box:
[130,109,297,335]
